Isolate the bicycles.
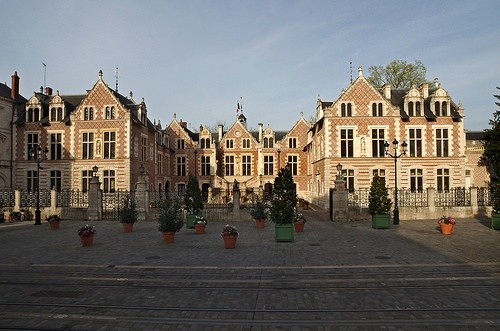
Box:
[9,207,27,224]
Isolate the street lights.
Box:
[29,144,50,225]
[384,139,408,225]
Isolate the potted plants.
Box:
[184,172,205,229]
[477,86,500,230]
[266,166,298,243]
[301,200,309,210]
[247,201,271,228]
[119,194,140,232]
[367,174,393,230]
[154,196,184,242]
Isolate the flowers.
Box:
[293,213,307,224]
[46,214,64,222]
[437,215,456,225]
[221,223,240,239]
[299,199,305,202]
[77,223,98,237]
[192,216,208,228]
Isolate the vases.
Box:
[439,223,454,235]
[193,224,205,234]
[80,234,95,247]
[294,222,305,232]
[222,235,238,249]
[299,201,304,206]
[50,220,61,231]
